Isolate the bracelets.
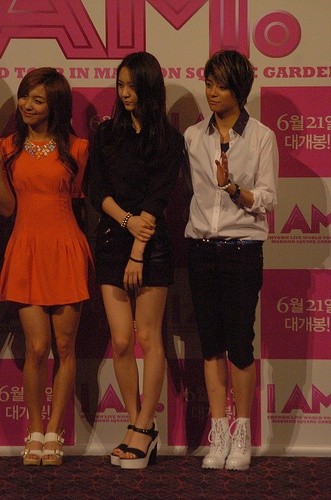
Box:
[128,255,144,263]
[217,179,231,191]
[230,183,241,204]
[121,211,133,230]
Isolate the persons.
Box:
[81,51,192,470]
[0,67,95,465]
[184,48,278,469]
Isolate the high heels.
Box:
[111,423,136,465]
[118,427,159,470]
[42,428,65,465]
[21,432,45,466]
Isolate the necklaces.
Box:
[22,134,57,158]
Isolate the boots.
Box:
[201,416,232,469]
[224,418,251,470]
